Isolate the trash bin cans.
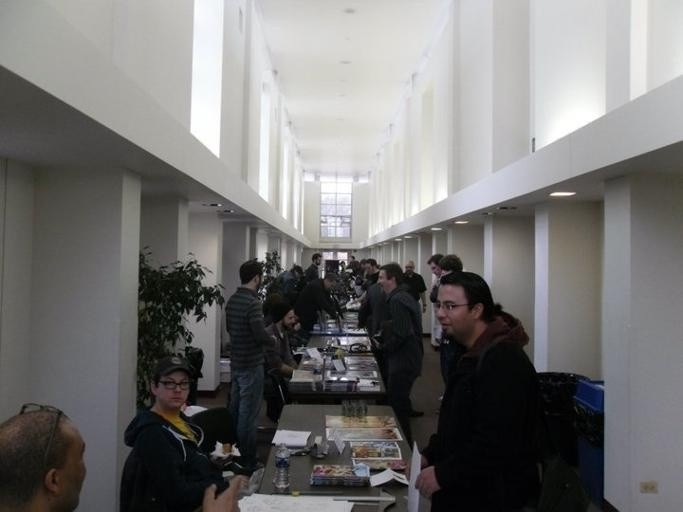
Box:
[537,371,590,466]
[575,380,605,509]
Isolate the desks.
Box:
[258,405,431,512]
[286,335,386,401]
[311,310,368,336]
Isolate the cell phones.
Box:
[216,477,229,499]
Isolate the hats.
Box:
[270,302,292,323]
[152,356,191,376]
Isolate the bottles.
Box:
[275,442,291,489]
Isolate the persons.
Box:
[0,401,247,512]
[124,356,255,512]
[224,255,427,447]
[427,256,465,400]
[415,271,541,512]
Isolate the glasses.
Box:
[404,266,412,270]
[21,402,63,468]
[159,381,191,390]
[432,302,469,310]
